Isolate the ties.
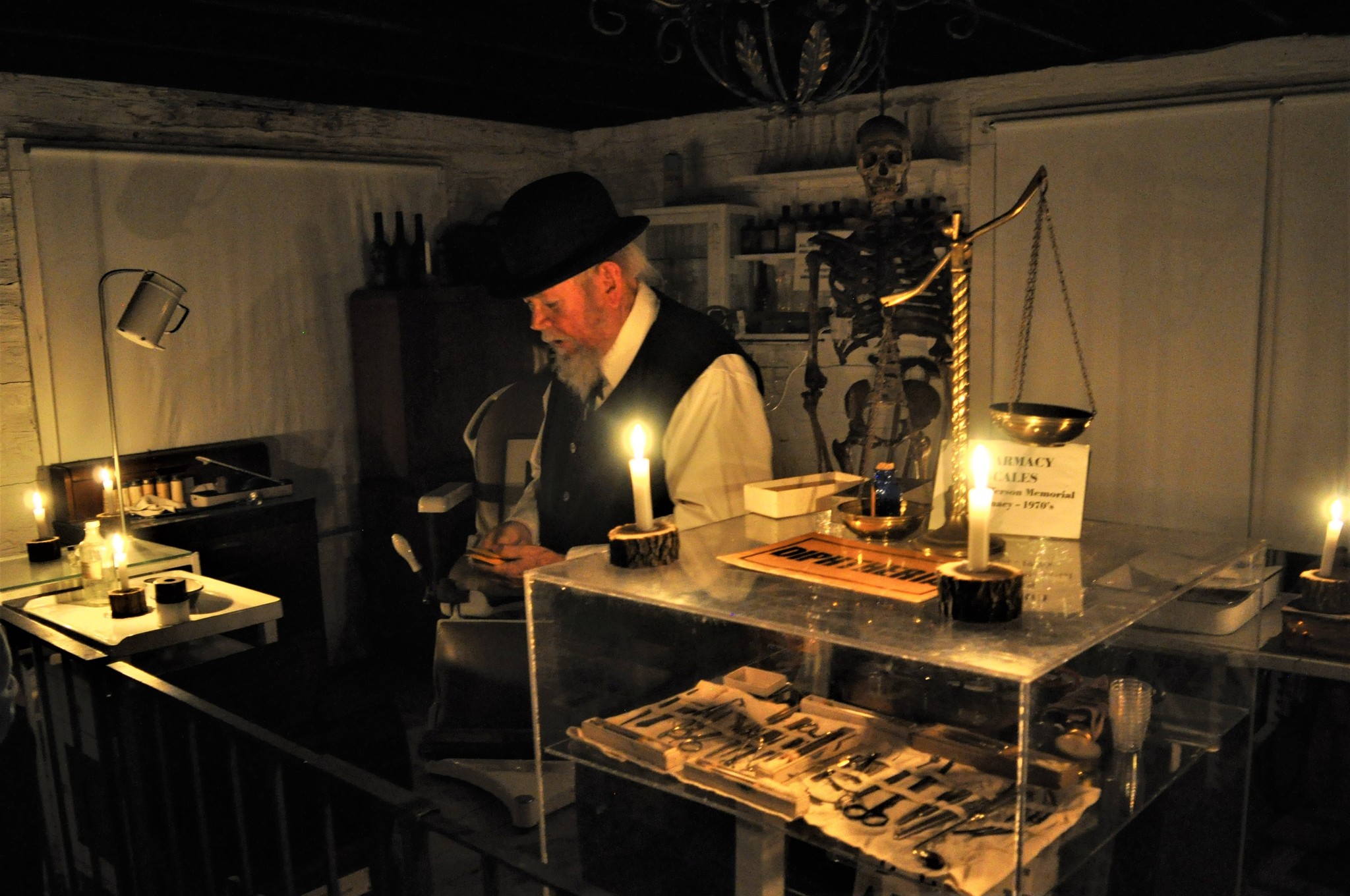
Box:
[583,376,606,416]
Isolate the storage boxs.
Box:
[744,470,870,518]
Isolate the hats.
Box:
[487,171,650,299]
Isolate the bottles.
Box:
[78,521,118,606]
[870,462,902,516]
[154,576,190,627]
[102,477,184,511]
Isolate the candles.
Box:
[1321,520,1344,578]
[118,558,129,591]
[629,457,654,531]
[33,508,47,540]
[103,480,116,515]
[968,488,994,572]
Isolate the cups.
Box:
[1108,678,1153,754]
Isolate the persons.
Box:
[467,171,772,896]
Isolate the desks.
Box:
[1,534,573,896]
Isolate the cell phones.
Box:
[466,547,505,566]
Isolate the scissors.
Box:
[667,704,802,753]
[842,758,955,827]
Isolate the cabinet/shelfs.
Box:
[1107,621,1350,896]
[354,287,552,623]
[525,496,1272,896]
[634,152,953,478]
[50,438,325,640]
